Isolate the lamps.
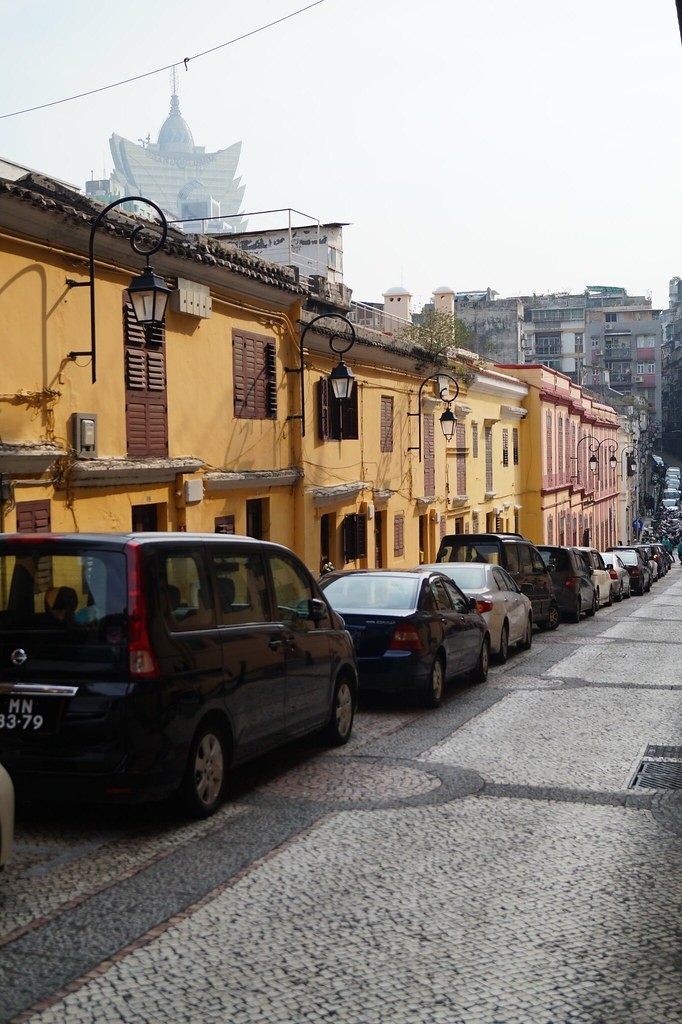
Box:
[617,446,639,477]
[598,438,618,480]
[66,197,171,384]
[634,419,665,465]
[407,373,459,463]
[283,313,355,437]
[570,436,600,478]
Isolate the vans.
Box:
[435,533,561,630]
[1,534,358,813]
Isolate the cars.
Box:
[611,550,652,595]
[665,467,681,490]
[314,567,491,705]
[606,545,654,585]
[600,553,631,601]
[659,499,681,512]
[664,490,681,500]
[636,543,675,582]
[415,562,533,664]
[653,457,664,466]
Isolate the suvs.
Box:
[578,547,613,611]
[534,545,598,622]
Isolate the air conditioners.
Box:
[605,323,614,330]
[595,349,603,356]
[635,376,644,382]
[525,350,533,355]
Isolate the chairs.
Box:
[214,577,236,613]
[167,585,181,613]
[44,586,78,625]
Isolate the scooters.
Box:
[641,508,682,543]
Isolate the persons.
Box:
[657,464,666,477]
[626,506,682,565]
[644,493,654,515]
